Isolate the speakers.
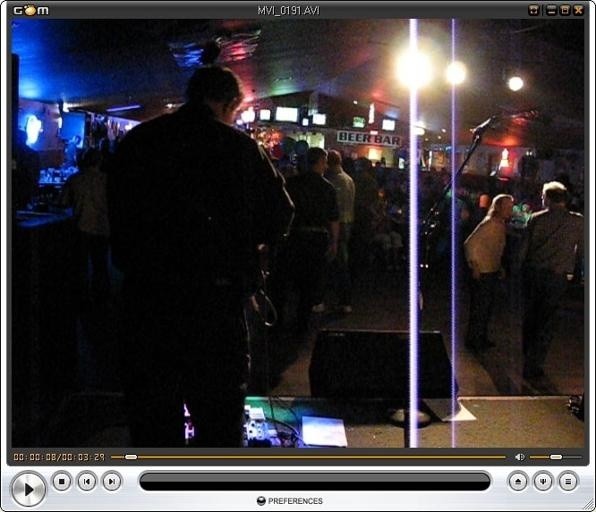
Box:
[309,329,458,399]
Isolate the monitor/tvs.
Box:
[0,0,595,512]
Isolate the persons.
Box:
[102,65,295,447]
[59,133,584,393]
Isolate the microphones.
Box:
[522,110,539,119]
